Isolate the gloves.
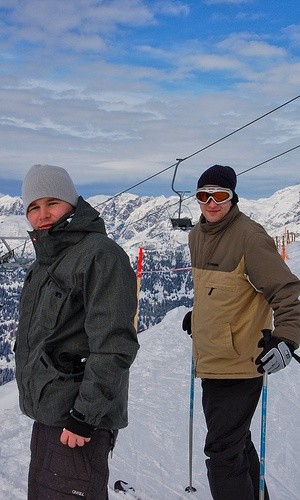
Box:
[182,312,193,338]
[256,337,300,375]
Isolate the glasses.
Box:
[194,186,233,204]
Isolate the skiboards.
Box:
[113,478,147,498]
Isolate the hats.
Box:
[21,165,79,228]
[198,165,238,203]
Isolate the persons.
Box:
[12,163,141,495]
[182,166,299,496]
